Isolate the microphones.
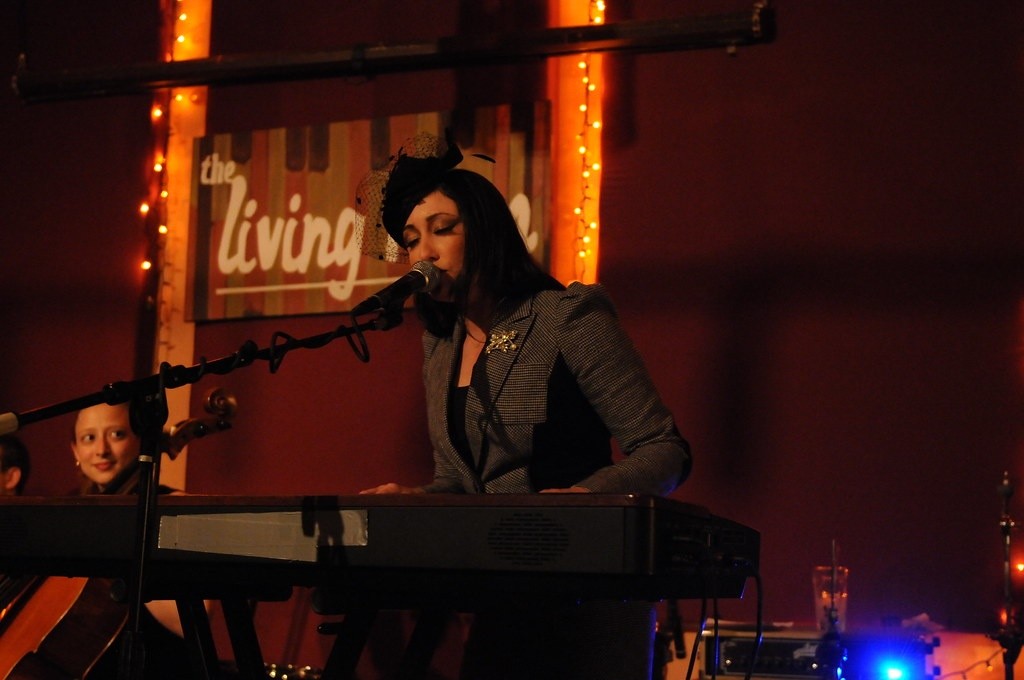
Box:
[351,261,441,316]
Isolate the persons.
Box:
[359,129,693,680]
[71,403,212,635]
[0,433,31,496]
